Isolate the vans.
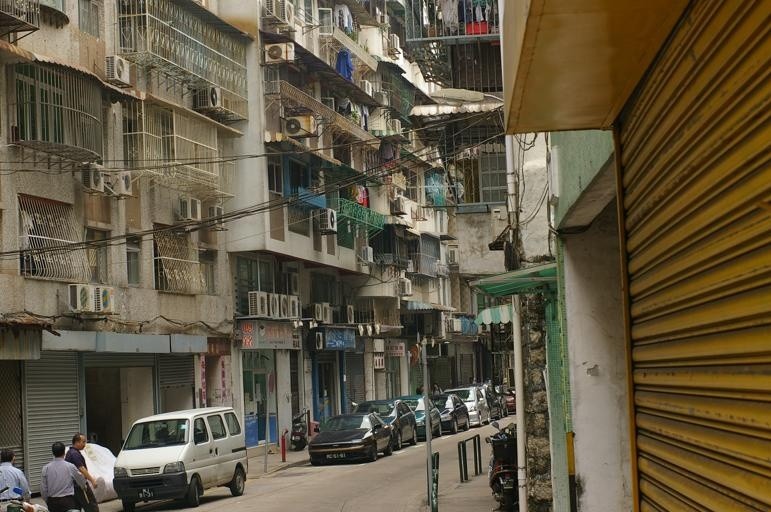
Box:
[113,406,249,511]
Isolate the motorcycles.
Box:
[291,407,308,452]
[485,421,520,511]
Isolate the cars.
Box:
[428,393,471,434]
[391,395,443,441]
[309,411,394,466]
[442,386,491,426]
[352,399,418,450]
[457,379,517,420]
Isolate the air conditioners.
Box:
[447,318,461,334]
[397,276,413,297]
[80,163,104,191]
[207,205,228,232]
[92,285,113,313]
[178,196,201,223]
[102,55,130,87]
[447,247,463,267]
[109,168,131,197]
[247,292,354,353]
[263,1,432,269]
[195,84,220,110]
[66,284,96,314]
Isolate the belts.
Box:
[0,499,24,502]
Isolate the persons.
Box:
[416,384,426,395]
[432,383,443,396]
[0,449,32,503]
[41,441,87,511]
[65,432,100,511]
